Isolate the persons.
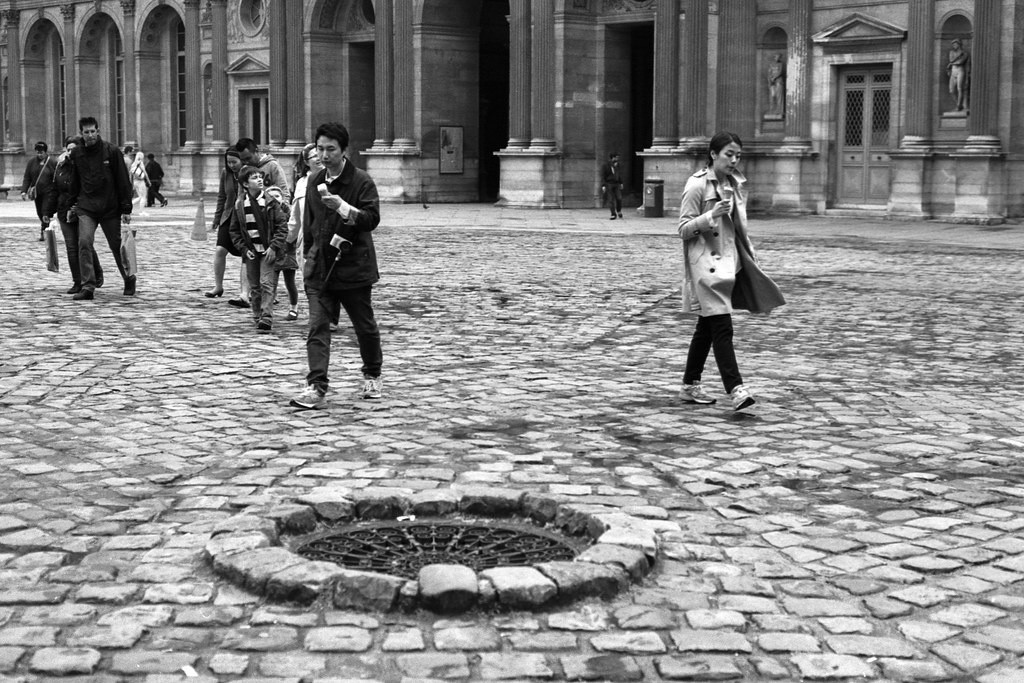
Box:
[41,136,104,294]
[144,153,168,207]
[206,79,212,118]
[123,146,151,216]
[288,122,383,410]
[73,116,137,300]
[767,53,784,115]
[601,152,623,220]
[285,143,341,332]
[20,141,58,242]
[678,132,786,410]
[264,186,299,321]
[228,166,288,334]
[204,146,243,297]
[946,38,969,111]
[228,138,289,308]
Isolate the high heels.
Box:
[205,288,223,298]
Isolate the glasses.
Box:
[306,154,318,160]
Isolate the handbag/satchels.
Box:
[22,186,37,201]
[119,220,138,276]
[42,229,59,272]
[131,162,145,179]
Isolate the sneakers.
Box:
[357,375,383,398]
[289,384,328,410]
[731,385,755,410]
[679,381,717,403]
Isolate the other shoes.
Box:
[258,319,272,330]
[145,204,152,208]
[228,297,251,308]
[67,284,81,294]
[160,199,168,207]
[330,322,337,331]
[273,299,279,304]
[123,275,136,296]
[96,269,103,288]
[285,310,298,320]
[73,289,93,300]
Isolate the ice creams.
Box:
[724,186,733,200]
[317,183,329,196]
